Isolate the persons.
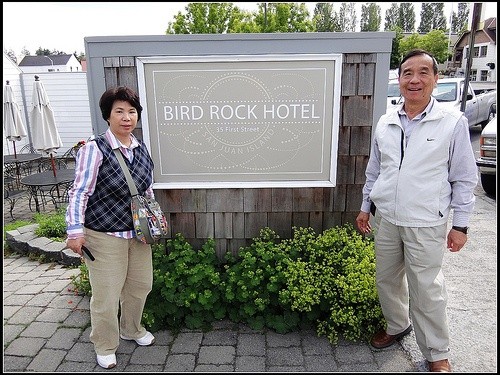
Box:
[355,48,479,372]
[65,85,156,370]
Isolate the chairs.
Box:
[4,145,75,220]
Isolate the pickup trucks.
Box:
[432,78,497,126]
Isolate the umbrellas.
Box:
[3,80,28,175]
[29,75,63,198]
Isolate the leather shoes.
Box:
[429,359,451,372]
[370,324,413,349]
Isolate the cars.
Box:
[386,70,405,117]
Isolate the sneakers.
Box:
[120,331,155,345]
[97,353,117,369]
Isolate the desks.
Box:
[21,169,75,214]
[3,153,42,185]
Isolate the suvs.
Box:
[476,114,496,197]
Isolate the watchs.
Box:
[452,225,467,234]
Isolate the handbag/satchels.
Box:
[131,195,168,244]
